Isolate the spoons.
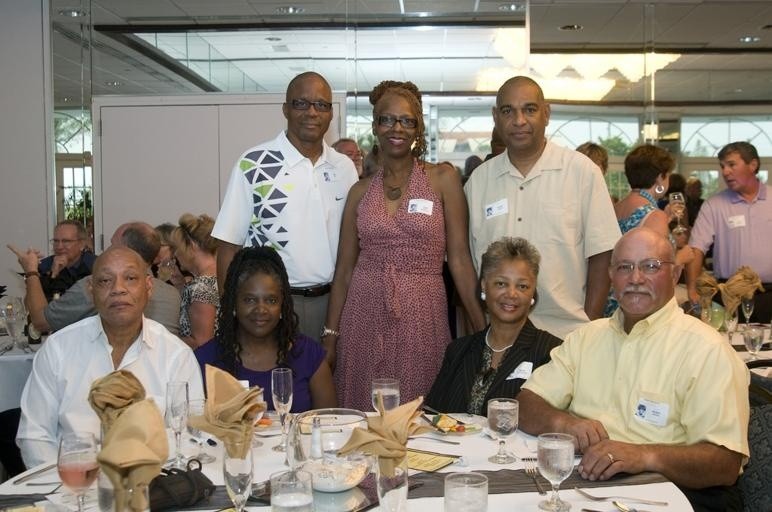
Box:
[613,501,648,512]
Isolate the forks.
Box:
[574,487,668,505]
[523,457,547,496]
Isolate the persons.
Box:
[510,226,752,512]
[192,244,341,415]
[414,235,564,418]
[210,71,626,414]
[6,214,226,348]
[13,244,205,471]
[571,139,772,326]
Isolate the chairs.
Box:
[1,407,26,476]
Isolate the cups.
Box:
[375,447,408,511]
[700,304,724,331]
[269,471,315,512]
[371,379,400,412]
[443,473,488,512]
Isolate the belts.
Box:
[289,284,332,299]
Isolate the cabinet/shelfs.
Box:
[91,92,347,256]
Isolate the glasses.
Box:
[377,115,418,130]
[50,238,81,248]
[287,98,333,113]
[613,257,675,275]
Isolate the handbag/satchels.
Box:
[150,469,216,510]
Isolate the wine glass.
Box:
[158,256,175,282]
[271,368,293,451]
[161,381,194,470]
[56,431,101,512]
[743,325,767,363]
[742,299,754,324]
[669,191,688,233]
[4,314,25,353]
[724,304,739,345]
[487,398,520,465]
[224,442,253,512]
[538,433,575,512]
